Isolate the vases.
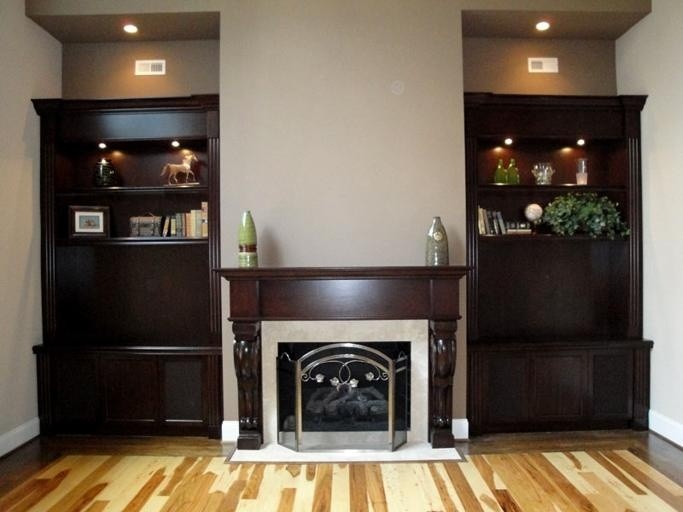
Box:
[236,210,259,268]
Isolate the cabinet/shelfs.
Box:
[466,89,648,436]
[25,92,221,443]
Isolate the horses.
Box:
[159,153,199,186]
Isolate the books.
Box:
[162,202,208,238]
[478,205,532,235]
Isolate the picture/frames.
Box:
[66,202,110,242]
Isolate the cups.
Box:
[575,157,589,186]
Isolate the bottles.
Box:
[92,157,116,187]
[238,212,259,267]
[493,158,520,185]
